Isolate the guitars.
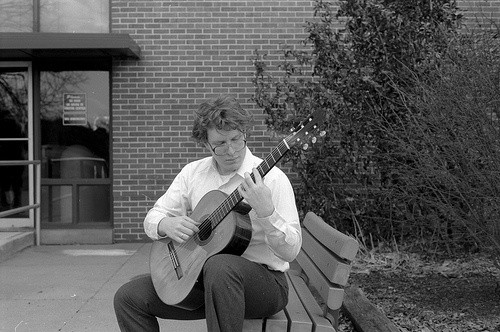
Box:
[149,109,327,310]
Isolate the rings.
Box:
[179,232,185,238]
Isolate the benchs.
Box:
[242,212,359,332]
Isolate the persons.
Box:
[113,93,302,332]
[0,108,28,215]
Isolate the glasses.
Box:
[207,137,246,156]
